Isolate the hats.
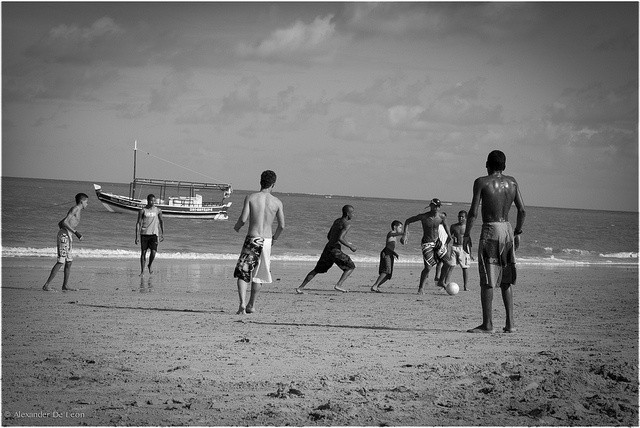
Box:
[431,199,441,206]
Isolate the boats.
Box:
[93,139,233,221]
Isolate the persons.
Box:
[399,198,458,296]
[462,149,527,335]
[434,211,449,281]
[371,219,409,293]
[295,204,357,295]
[134,193,165,279]
[40,192,89,292]
[232,169,286,315]
[443,209,475,292]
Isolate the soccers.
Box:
[446,282,459,295]
